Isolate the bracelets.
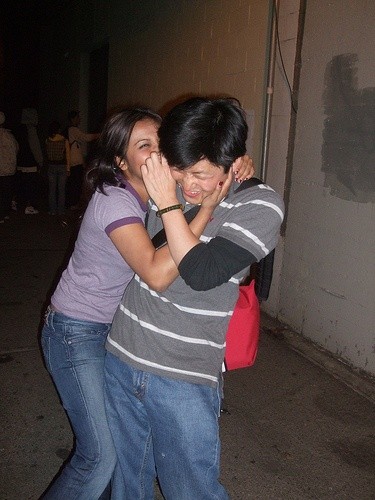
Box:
[155,204,185,217]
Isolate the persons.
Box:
[103,94,284,500]
[39,106,255,500]
[64,110,103,211]
[0,111,19,224]
[12,106,44,214]
[44,121,70,216]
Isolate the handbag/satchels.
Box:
[221,279,260,372]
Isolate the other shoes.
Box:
[25,207,39,214]
